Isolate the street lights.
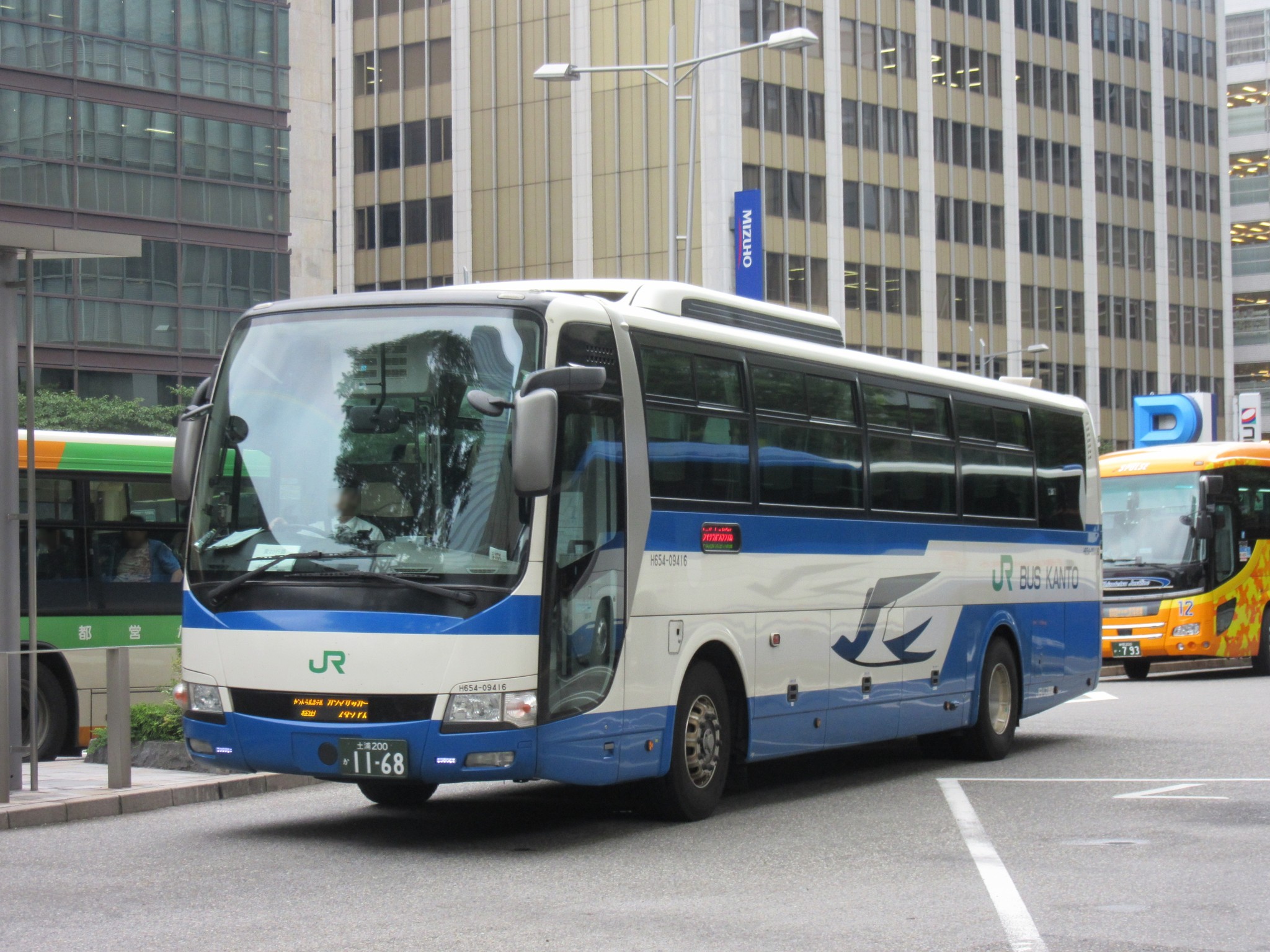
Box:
[533,25,819,288]
[979,343,1049,378]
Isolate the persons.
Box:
[268,476,385,553]
[106,515,184,581]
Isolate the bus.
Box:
[1077,439,1270,679]
[18,429,433,768]
[171,277,1104,821]
